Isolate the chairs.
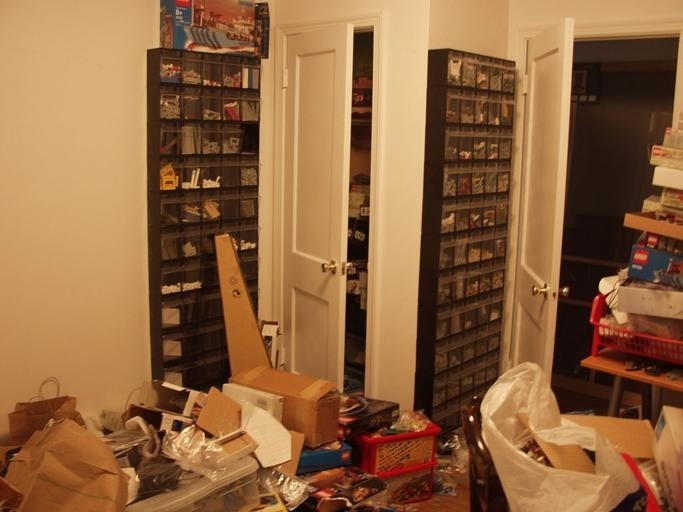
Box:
[461,395,496,512]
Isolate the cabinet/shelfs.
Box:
[344,26,374,393]
[148,46,262,389]
[552,255,642,407]
[413,48,517,435]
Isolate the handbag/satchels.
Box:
[7,376,87,445]
[478,359,649,511]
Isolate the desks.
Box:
[581,356,683,428]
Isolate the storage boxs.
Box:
[650,406,683,512]
[124,455,261,512]
[227,363,340,450]
